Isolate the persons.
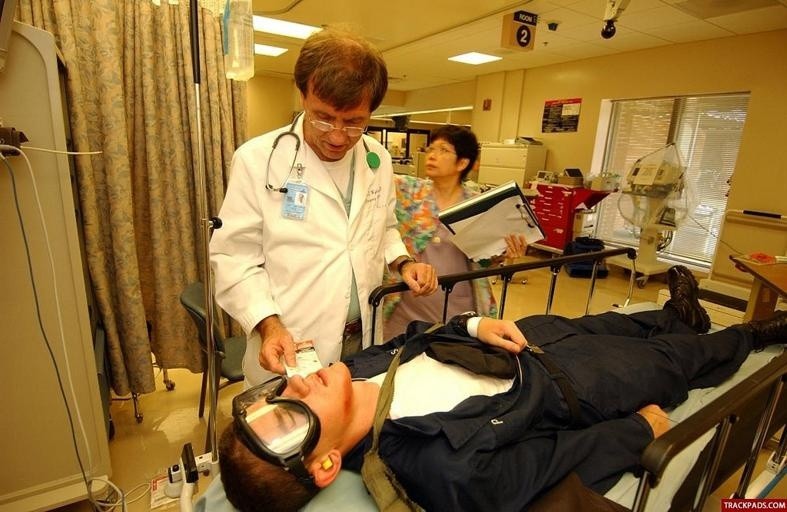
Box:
[381,124,527,345]
[208,23,439,394]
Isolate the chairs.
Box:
[177,281,248,456]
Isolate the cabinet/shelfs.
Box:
[478,142,548,191]
[522,181,585,270]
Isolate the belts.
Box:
[342,319,362,340]
[524,342,583,422]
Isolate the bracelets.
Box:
[396,258,417,276]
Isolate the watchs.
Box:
[458,311,479,332]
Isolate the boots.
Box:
[663,264,711,332]
[732,310,786,350]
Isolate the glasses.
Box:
[422,146,457,155]
[307,114,368,136]
[232,377,319,470]
